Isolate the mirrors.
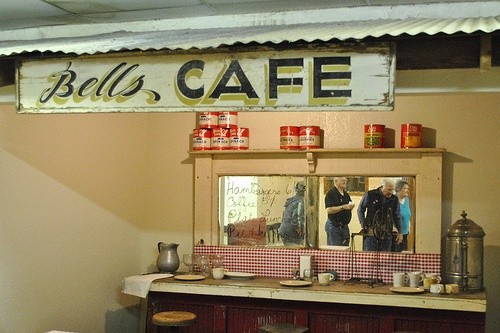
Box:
[217,174,307,248]
[315,175,417,253]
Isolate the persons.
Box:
[280,176,411,251]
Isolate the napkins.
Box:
[122,274,173,299]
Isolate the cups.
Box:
[393,273,405,287]
[408,272,424,287]
[445,283,459,294]
[304,269,317,285]
[424,272,441,292]
[430,284,444,294]
[212,268,228,279]
[318,273,334,285]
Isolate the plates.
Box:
[390,287,425,293]
[224,271,256,280]
[280,280,312,286]
[174,274,205,281]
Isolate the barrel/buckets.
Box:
[445,211,485,290]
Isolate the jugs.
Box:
[157,242,180,274]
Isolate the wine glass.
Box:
[184,254,194,272]
[195,254,224,274]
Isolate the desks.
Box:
[128,271,487,333]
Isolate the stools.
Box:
[261,323,307,333]
[152,311,197,333]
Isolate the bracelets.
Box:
[340,205,344,210]
